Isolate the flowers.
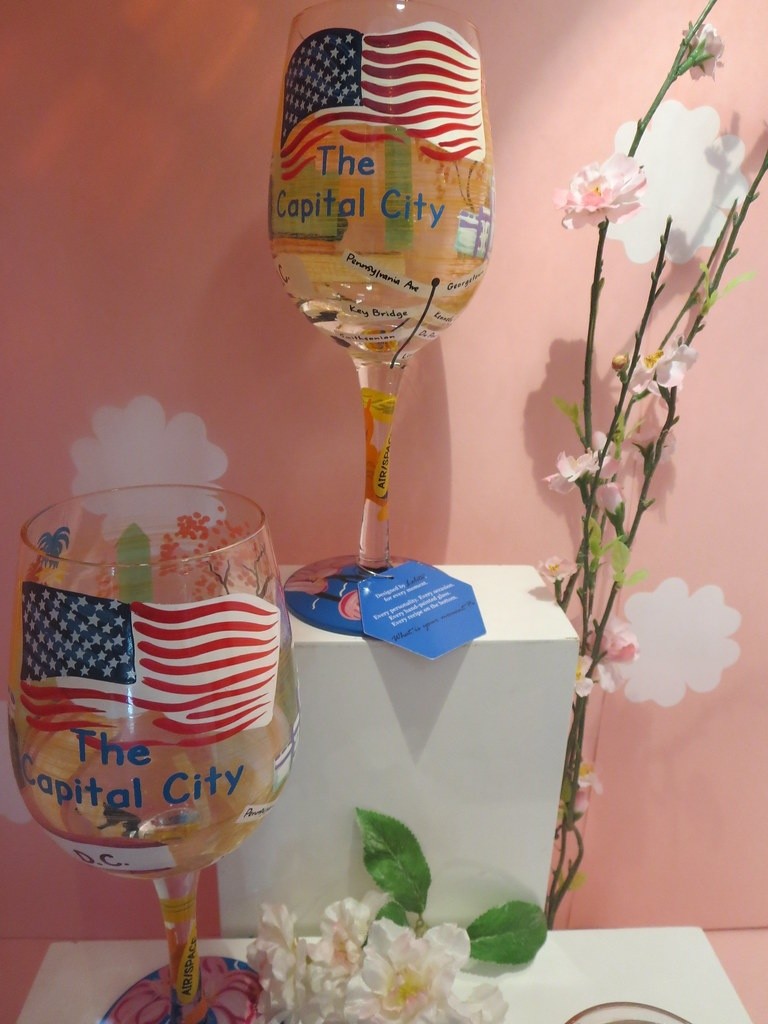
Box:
[238,806,551,1023]
[544,0,768,937]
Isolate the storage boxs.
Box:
[209,556,580,944]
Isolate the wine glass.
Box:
[6,484,300,1024]
[269,1,491,635]
